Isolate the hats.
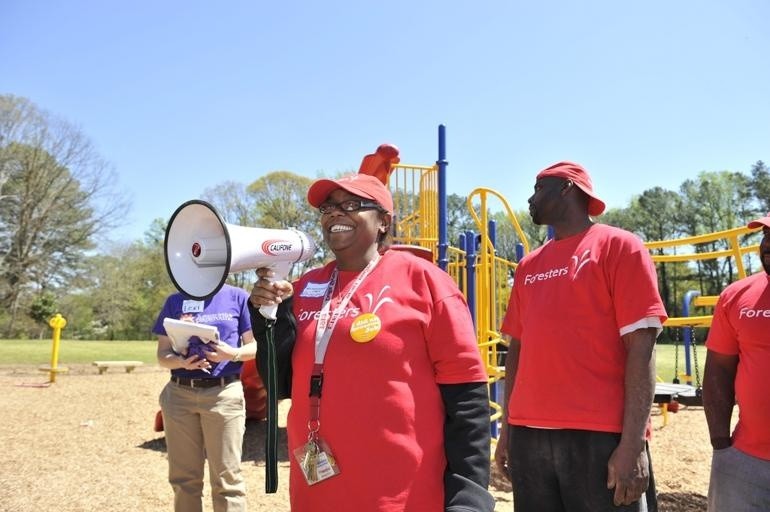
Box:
[747,209,770,232]
[536,161,604,216]
[307,174,393,216]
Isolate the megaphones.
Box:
[164,199,314,320]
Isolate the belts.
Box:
[170,374,241,389]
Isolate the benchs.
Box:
[92,360,144,375]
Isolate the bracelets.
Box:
[232,350,240,363]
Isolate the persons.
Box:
[152,279,251,512]
[246,173,497,512]
[495,161,660,512]
[702,209,769,511]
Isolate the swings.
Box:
[673,326,702,406]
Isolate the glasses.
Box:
[319,198,381,215]
